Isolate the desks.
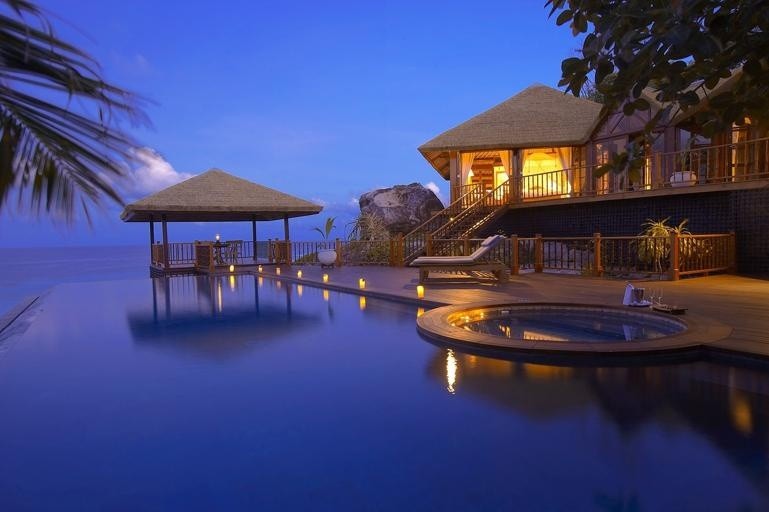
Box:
[212,244,231,265]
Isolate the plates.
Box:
[654,304,690,311]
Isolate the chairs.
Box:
[409,235,508,288]
[225,240,245,265]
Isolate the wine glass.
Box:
[649,287,663,309]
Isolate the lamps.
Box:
[229,265,235,272]
[229,275,235,288]
[258,277,366,310]
[215,234,220,244]
[416,285,425,300]
[257,265,366,290]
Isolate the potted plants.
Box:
[310,215,338,264]
[670,120,718,188]
